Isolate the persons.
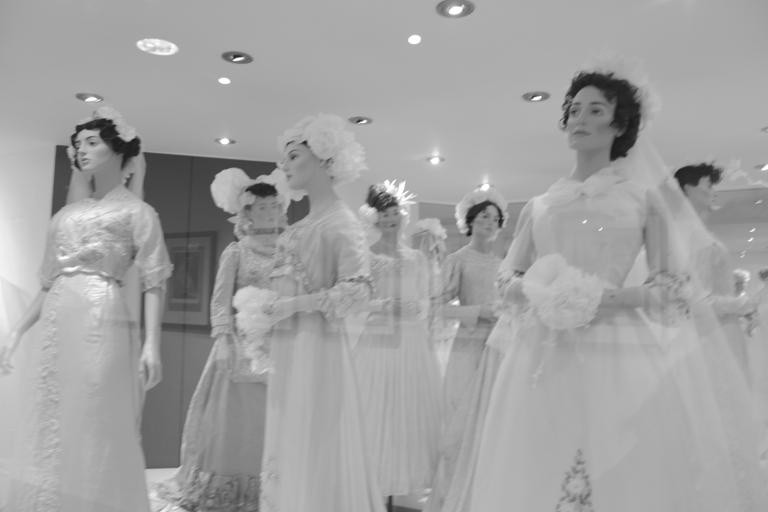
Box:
[665,159,768,399]
[361,181,441,512]
[434,182,509,512]
[204,164,292,512]
[404,216,456,374]
[0,105,174,512]
[231,109,381,512]
[492,73,768,512]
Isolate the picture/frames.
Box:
[161,231,219,336]
